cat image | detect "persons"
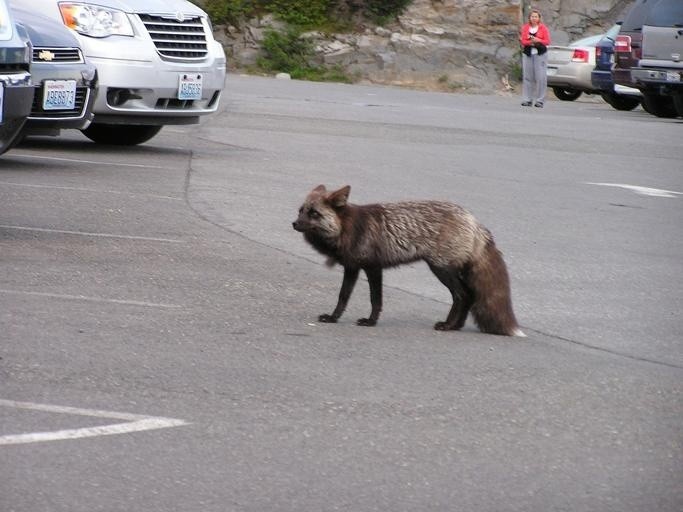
[519,10,551,108]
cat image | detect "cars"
[546,0,682,118]
[0,0,226,157]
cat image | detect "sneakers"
[521,101,544,108]
